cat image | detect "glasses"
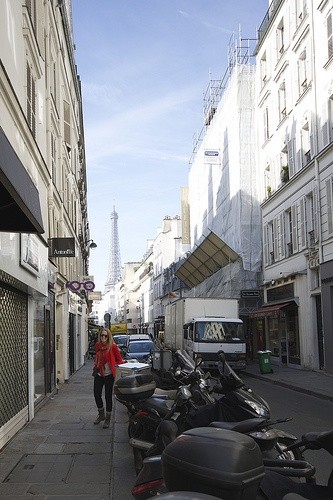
[101,335,108,337]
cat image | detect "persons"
[93,328,124,428]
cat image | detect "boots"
[102,413,111,429]
[93,406,105,425]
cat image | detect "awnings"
[254,301,295,317]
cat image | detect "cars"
[113,334,157,360]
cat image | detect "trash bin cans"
[257,350,273,374]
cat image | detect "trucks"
[164,296,247,375]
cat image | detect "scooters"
[114,348,333,500]
